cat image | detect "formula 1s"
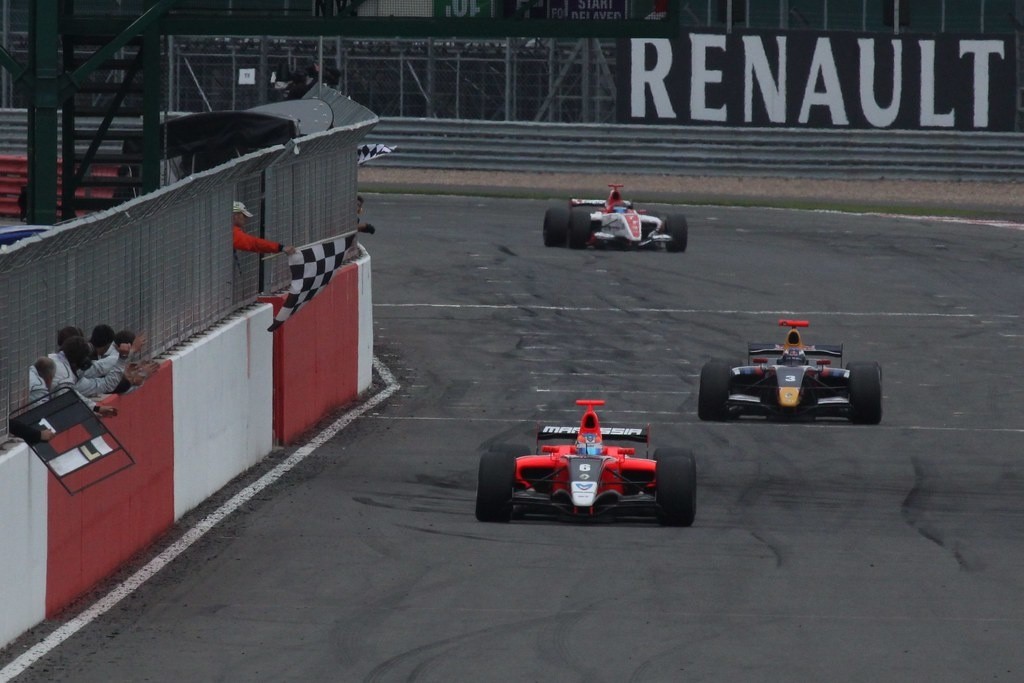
[542,181,690,255]
[690,318,886,425]
[473,399,702,529]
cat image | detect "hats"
[232,201,253,217]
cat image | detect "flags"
[266,230,360,332]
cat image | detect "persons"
[114,330,161,377]
[56,326,94,372]
[47,335,119,418]
[358,194,376,234]
[86,324,145,393]
[9,418,56,442]
[28,356,57,410]
[613,200,627,213]
[574,434,602,456]
[233,199,296,256]
[777,347,806,365]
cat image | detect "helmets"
[608,201,628,213]
[576,433,603,456]
[784,347,807,365]
